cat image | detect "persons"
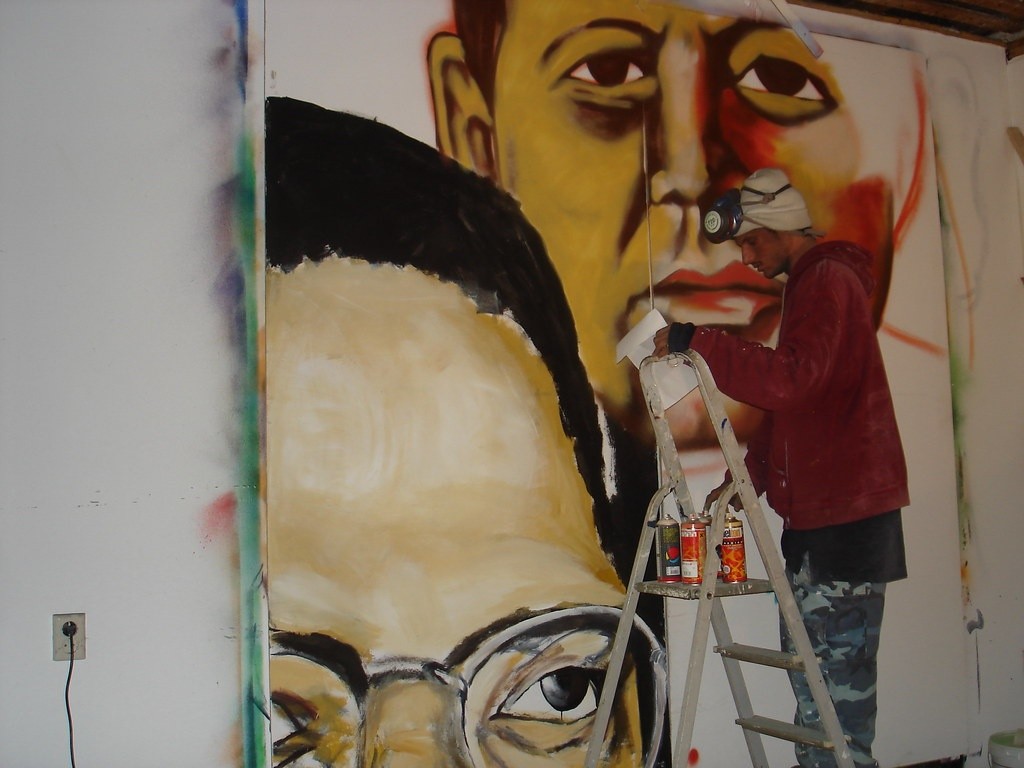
[650,168,911,768]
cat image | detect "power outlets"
[52,613,86,661]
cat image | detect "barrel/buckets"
[987,729,1024,768]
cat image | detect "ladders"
[583,349,856,768]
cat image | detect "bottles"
[721,513,748,584]
[680,510,722,585]
[654,513,682,584]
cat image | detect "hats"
[732,169,812,237]
